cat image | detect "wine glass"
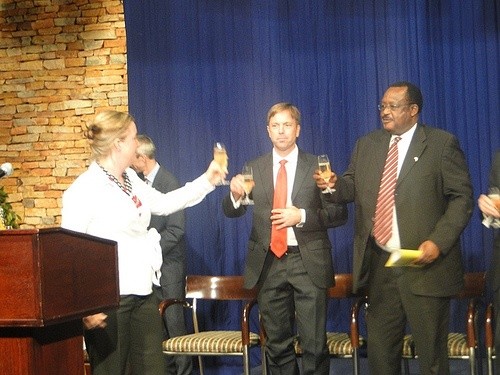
[213,142,229,186]
[317,155,336,194]
[487,187,500,228]
[240,166,254,205]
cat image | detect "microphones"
[0,163,13,178]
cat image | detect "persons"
[477,150,500,375]
[131,135,193,374]
[313,82,474,375]
[222,102,349,375]
[60,111,229,375]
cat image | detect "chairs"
[158,274,500,375]
